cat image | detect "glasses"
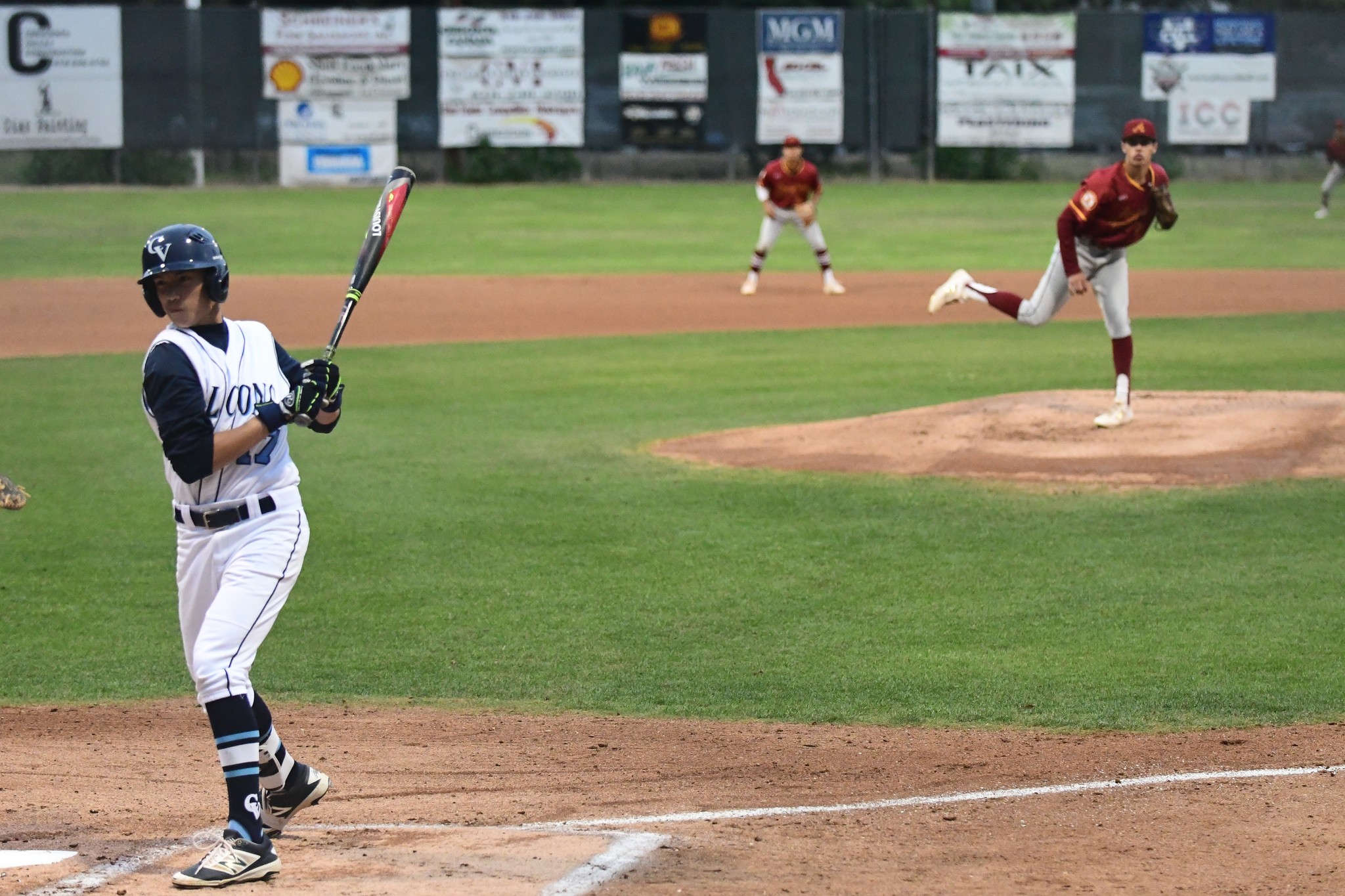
[1127,138,1151,146]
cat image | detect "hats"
[1124,119,1156,142]
[785,136,800,148]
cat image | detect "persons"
[136,223,343,888]
[1313,120,1345,218]
[739,136,847,295]
[0,474,30,510]
[929,119,1177,429]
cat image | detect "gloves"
[254,374,330,433]
[300,360,344,412]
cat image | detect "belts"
[175,496,275,529]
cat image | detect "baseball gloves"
[1150,184,1178,229]
[0,475,27,510]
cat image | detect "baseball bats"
[293,163,416,427]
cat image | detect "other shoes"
[740,273,758,294]
[822,282,844,295]
[1317,207,1329,219]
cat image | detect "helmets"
[137,225,229,317]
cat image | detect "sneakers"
[172,830,282,888]
[928,270,972,315]
[258,763,331,836]
[1094,403,1133,427]
